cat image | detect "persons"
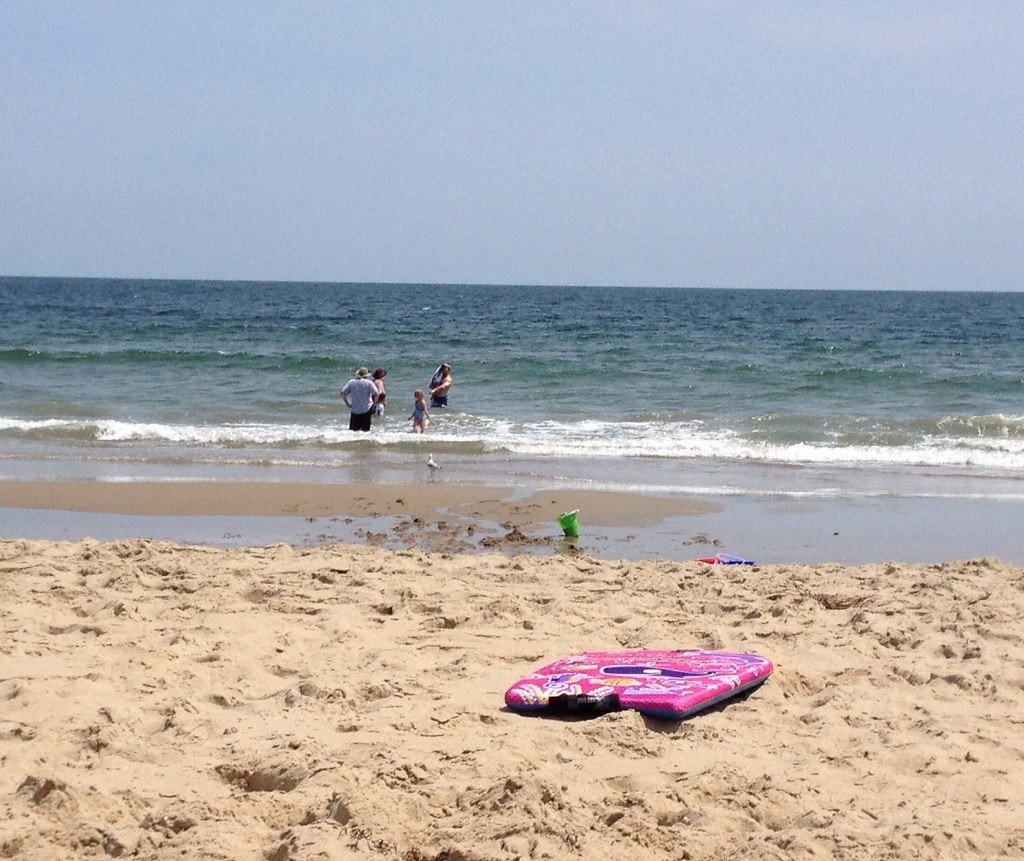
[375,393,386,416]
[341,367,379,431]
[372,368,387,394]
[429,362,453,408]
[408,389,434,434]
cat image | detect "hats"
[373,368,386,379]
[355,366,372,378]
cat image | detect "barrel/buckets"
[555,509,579,538]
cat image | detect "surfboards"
[502,646,775,723]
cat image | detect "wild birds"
[427,453,442,474]
